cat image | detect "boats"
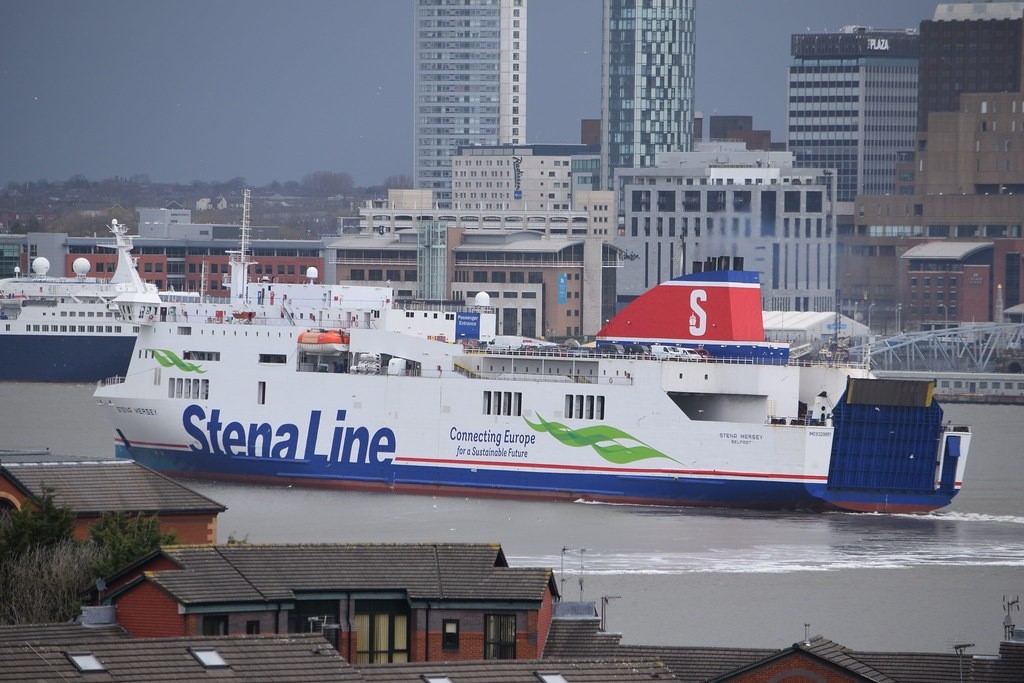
[89,184,973,519]
[0,216,166,380]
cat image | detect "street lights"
[898,303,903,334]
[895,307,901,337]
[868,302,876,330]
[937,304,949,352]
